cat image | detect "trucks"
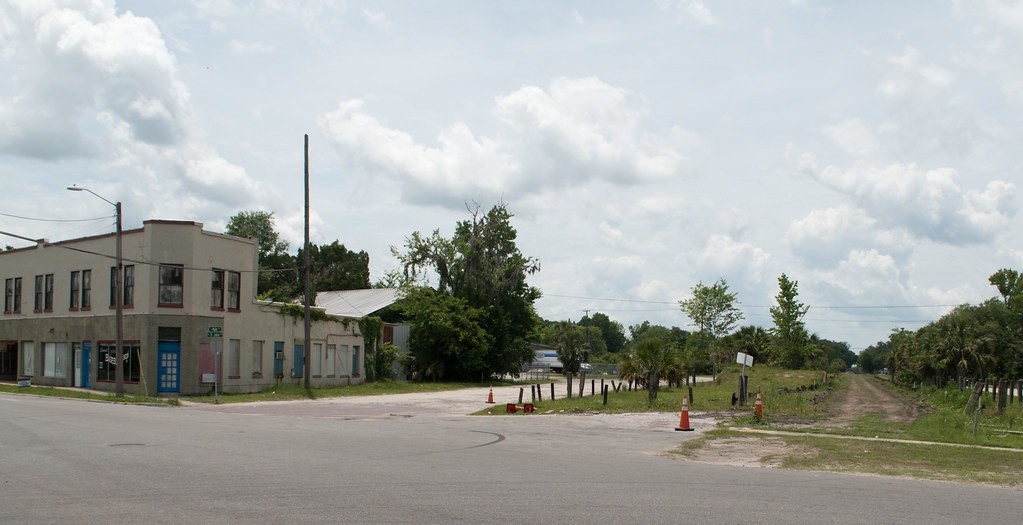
[879,368,890,374]
[527,348,593,373]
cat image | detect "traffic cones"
[505,403,524,412]
[485,384,495,403]
[753,390,764,418]
[522,404,538,414]
[674,393,694,432]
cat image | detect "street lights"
[68,184,125,397]
